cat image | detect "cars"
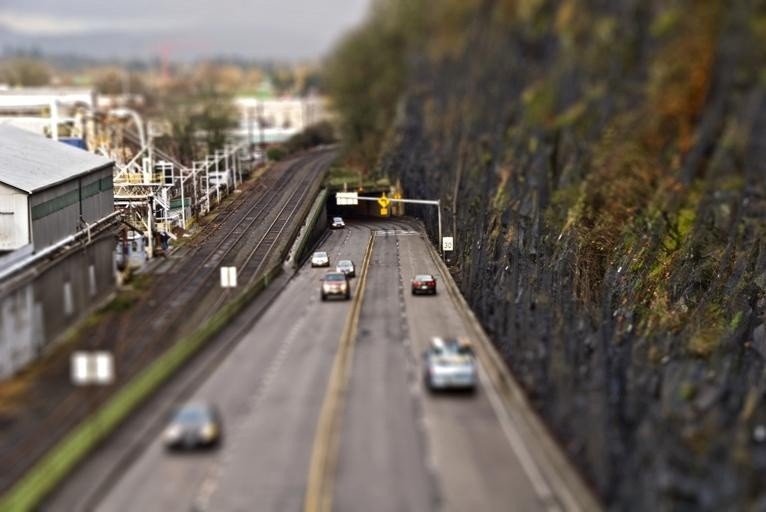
[319,271,350,299]
[164,402,218,453]
[424,339,476,394]
[411,274,436,294]
[331,216,346,229]
[334,259,355,276]
[311,251,329,268]
